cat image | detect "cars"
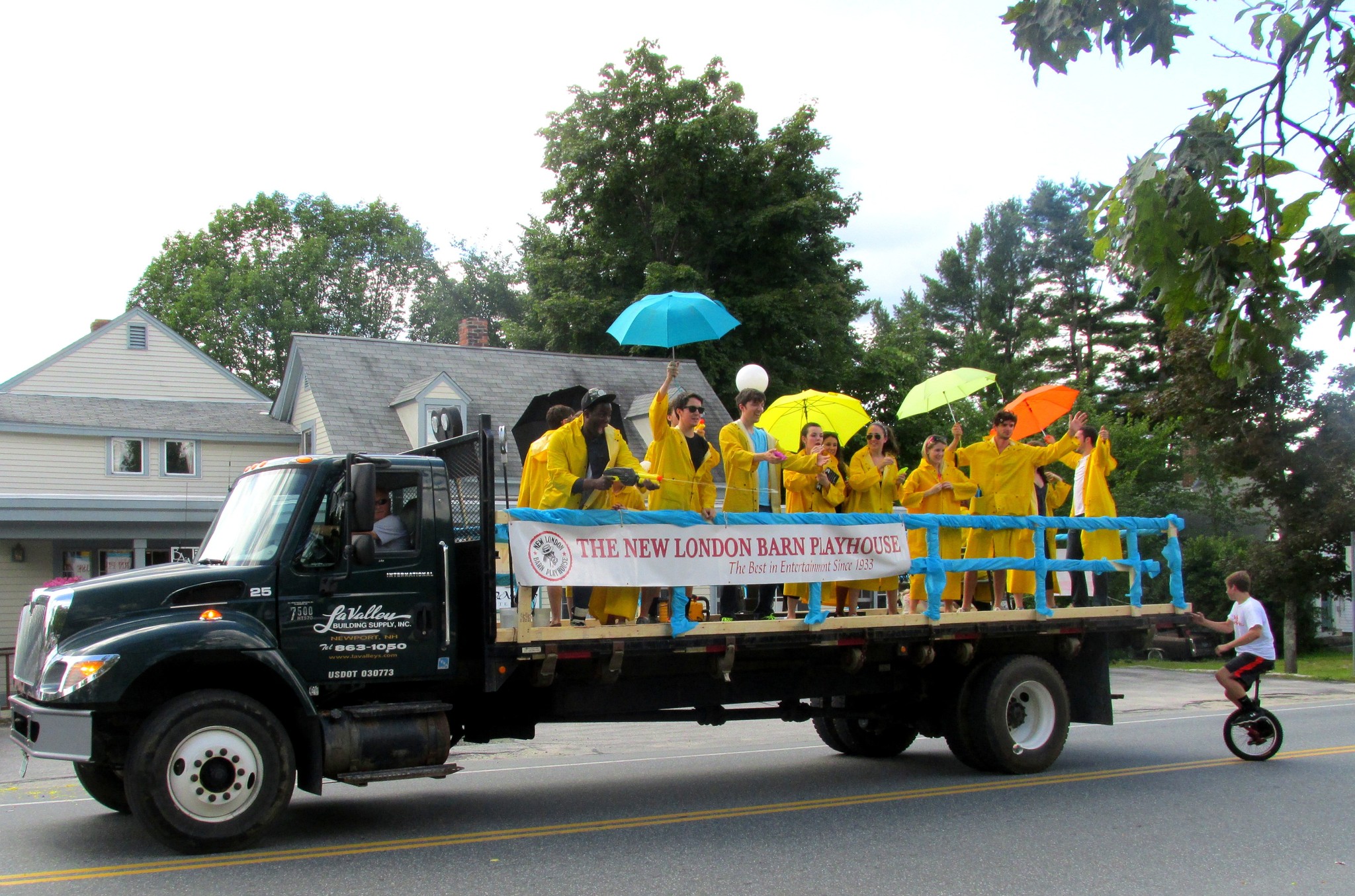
[1106,624,1217,662]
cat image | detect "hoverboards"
[1223,674,1283,762]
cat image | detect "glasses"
[682,406,705,414]
[866,435,885,440]
[926,436,947,445]
[823,431,838,437]
[375,498,390,505]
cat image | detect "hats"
[581,387,616,411]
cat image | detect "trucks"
[7,407,1196,855]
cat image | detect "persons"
[1189,570,1275,743]
[523,386,1125,626]
[309,488,411,554]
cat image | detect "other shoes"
[550,622,562,627]
[993,605,1008,610]
[887,611,900,615]
[849,613,857,616]
[957,608,966,612]
[1051,606,1059,608]
[721,617,733,622]
[635,614,655,624]
[1015,607,1024,610]
[1067,603,1074,608]
[762,613,777,620]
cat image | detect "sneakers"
[1247,725,1274,745]
[1231,709,1265,725]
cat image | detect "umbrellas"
[989,384,1080,442]
[607,291,741,384]
[895,367,1004,448]
[753,389,872,454]
